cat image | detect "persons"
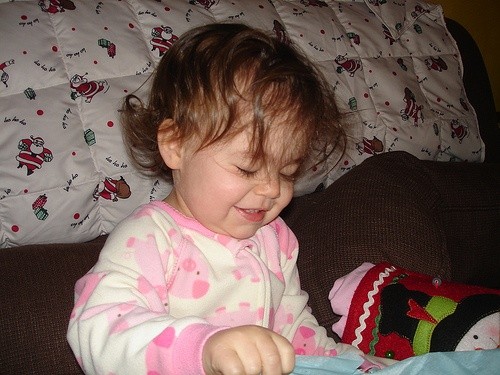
[66,20,383,375]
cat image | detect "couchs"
[1,18,500,375]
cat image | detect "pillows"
[285,148,451,345]
